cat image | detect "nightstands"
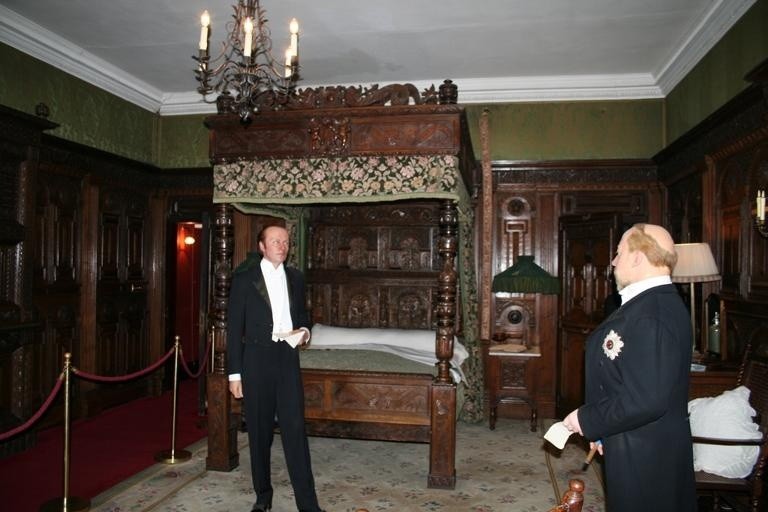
[485,347,544,431]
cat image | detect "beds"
[202,78,484,494]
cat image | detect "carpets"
[86,415,604,512]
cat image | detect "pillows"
[308,319,460,354]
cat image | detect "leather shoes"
[250,491,272,511]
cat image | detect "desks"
[675,363,739,400]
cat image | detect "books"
[488,344,527,352]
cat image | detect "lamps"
[490,252,559,351]
[664,241,722,352]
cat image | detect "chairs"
[675,319,767,510]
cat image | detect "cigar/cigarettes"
[585,445,597,465]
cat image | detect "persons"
[563,222,698,510]
[225,225,326,511]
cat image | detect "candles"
[284,47,294,77]
[753,186,767,224]
[289,16,300,56]
[199,7,209,52]
[243,14,254,56]
